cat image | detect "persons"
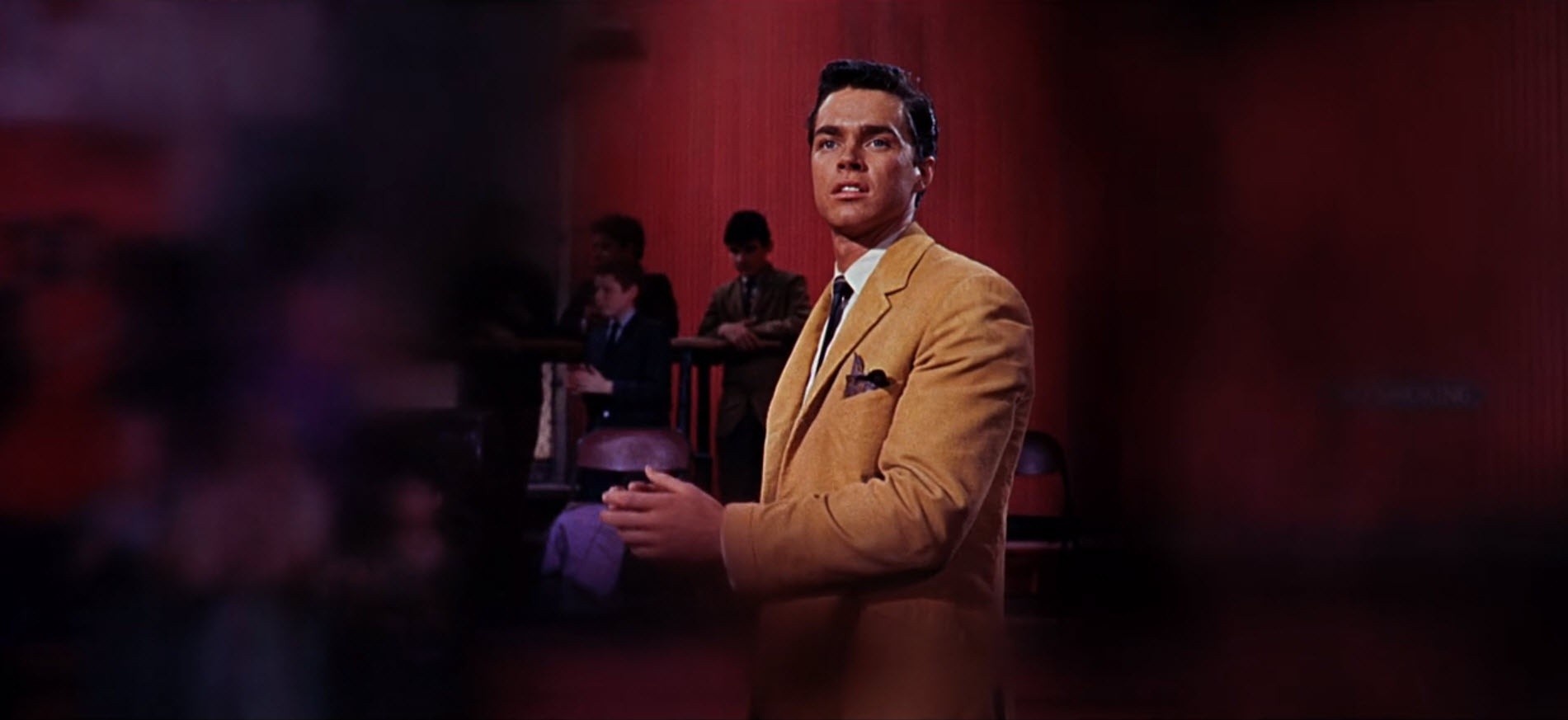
[598,60,1035,720]
[435,206,556,496]
[699,210,809,501]
[552,212,678,431]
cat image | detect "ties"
[608,318,622,347]
[817,275,852,375]
[744,281,755,315]
